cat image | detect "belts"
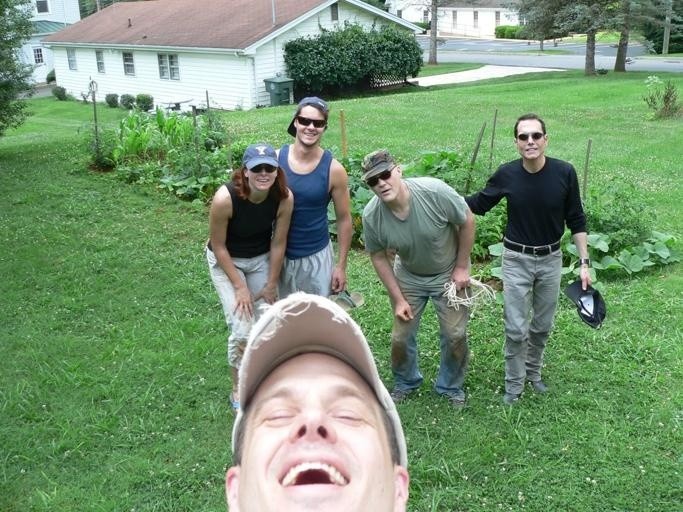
[502,239,561,257]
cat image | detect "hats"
[360,149,396,181]
[229,290,409,471]
[287,96,329,138]
[242,143,279,170]
[563,279,607,331]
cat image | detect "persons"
[273,95,353,310]
[361,147,475,403]
[205,143,294,411]
[225,291,411,511]
[460,113,593,405]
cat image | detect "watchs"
[579,258,590,264]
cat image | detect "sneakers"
[389,385,412,405]
[527,378,546,392]
[503,392,520,405]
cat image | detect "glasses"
[251,165,276,173]
[296,114,326,128]
[518,132,543,140]
[366,164,397,187]
